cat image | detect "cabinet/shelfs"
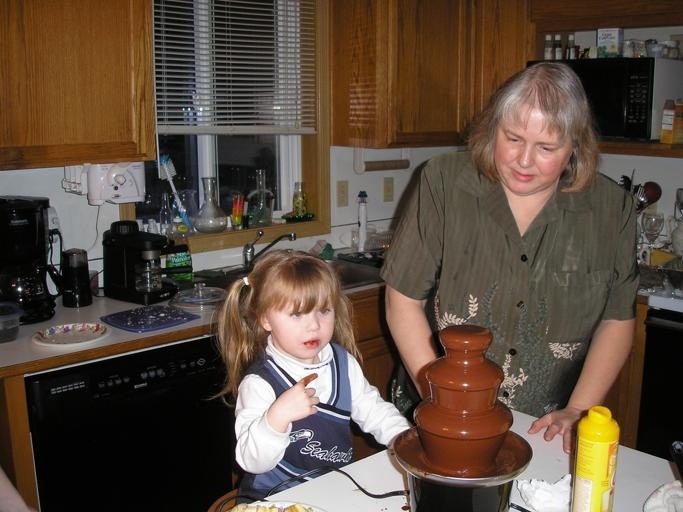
[602,294,650,448]
[330,0,532,149]
[0,0,155,171]
[533,1,683,158]
[331,283,401,459]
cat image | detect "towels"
[642,480,683,512]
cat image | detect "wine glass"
[640,210,665,251]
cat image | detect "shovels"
[636,180,661,212]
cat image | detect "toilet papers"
[358,202,367,252]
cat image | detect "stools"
[207,489,237,512]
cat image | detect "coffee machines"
[100,220,179,305]
[0,194,56,328]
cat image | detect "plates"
[31,322,110,348]
[283,213,315,223]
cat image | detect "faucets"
[243,230,296,268]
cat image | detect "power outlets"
[48,206,59,240]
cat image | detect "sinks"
[321,260,381,295]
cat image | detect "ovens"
[633,315,683,471]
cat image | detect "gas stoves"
[649,285,683,315]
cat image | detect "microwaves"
[526,58,683,144]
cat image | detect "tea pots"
[45,247,92,310]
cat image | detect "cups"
[352,230,377,251]
[230,194,244,229]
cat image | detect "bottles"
[291,181,308,218]
[623,37,683,58]
[147,218,159,235]
[196,174,227,233]
[159,193,173,236]
[251,170,273,225]
[544,33,577,60]
[173,189,198,235]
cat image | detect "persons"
[378,62,640,455]
[205,250,414,507]
